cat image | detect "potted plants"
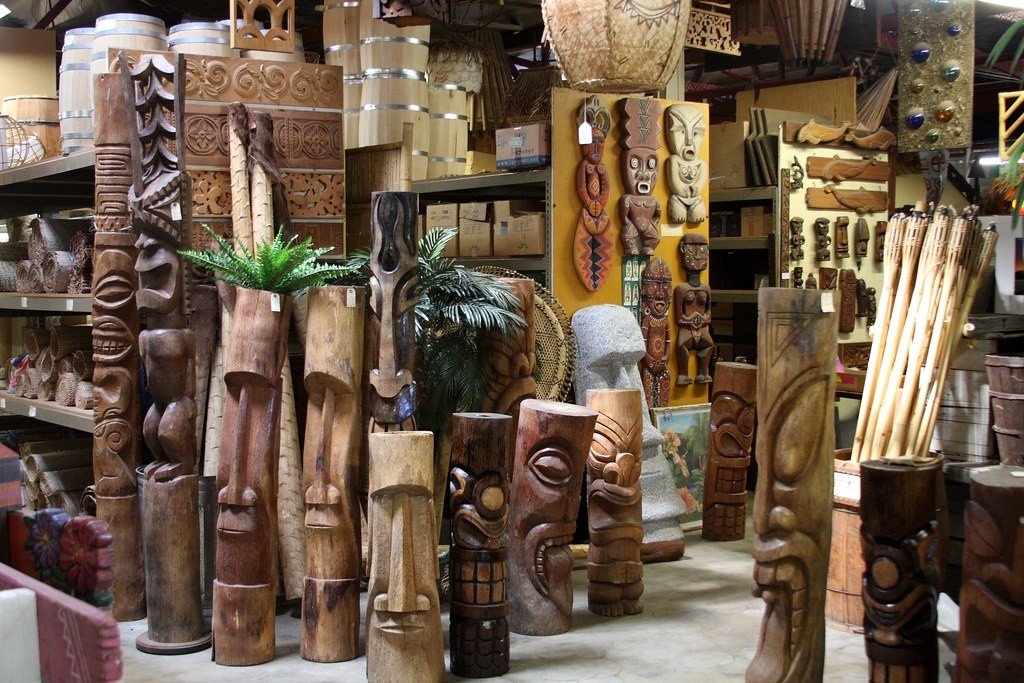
[176,218,353,666]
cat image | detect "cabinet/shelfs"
[0,151,96,435]
[710,185,780,302]
[411,168,551,292]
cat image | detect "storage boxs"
[495,123,551,170]
[709,206,773,361]
[493,200,547,257]
[457,202,495,257]
[426,203,458,258]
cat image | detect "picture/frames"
[649,402,712,532]
